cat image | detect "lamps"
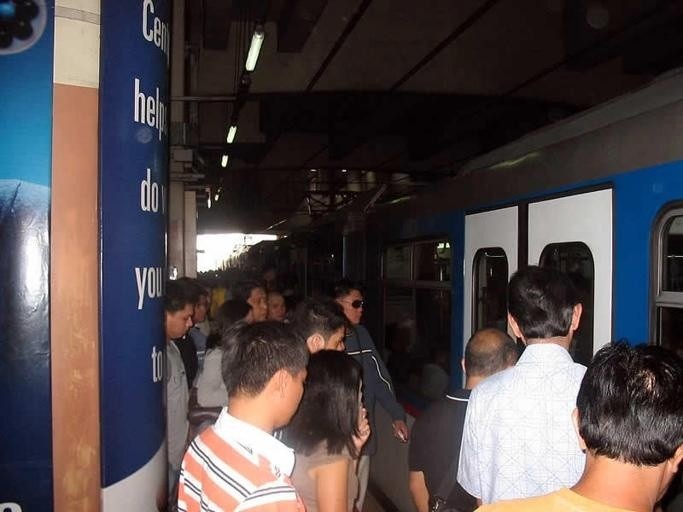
[221,27,265,170]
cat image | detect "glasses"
[340,299,365,307]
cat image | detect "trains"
[218,67,683,393]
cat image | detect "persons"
[410,266,683,512]
[164,262,409,511]
